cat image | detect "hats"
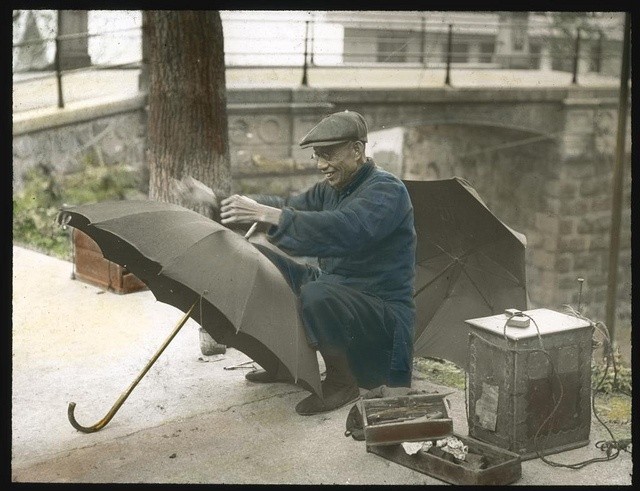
[299,111,368,149]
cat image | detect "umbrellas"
[57,199,325,434]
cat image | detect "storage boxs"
[72,228,151,295]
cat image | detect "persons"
[172,110,415,417]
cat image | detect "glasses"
[310,152,332,161]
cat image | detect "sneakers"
[295,376,361,416]
[245,367,288,382]
